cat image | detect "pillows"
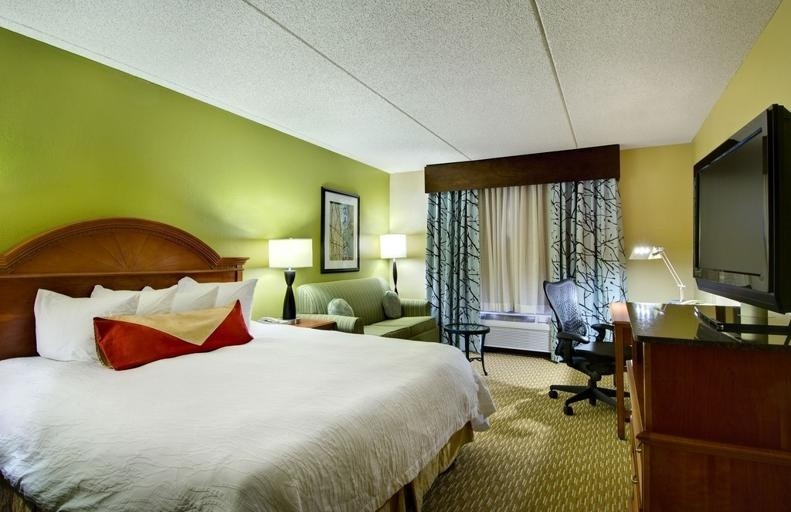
[327,298,354,316]
[382,290,402,319]
[32,277,257,363]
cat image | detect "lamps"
[629,244,697,306]
[268,238,313,320]
[380,235,407,298]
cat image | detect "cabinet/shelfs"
[629,304,791,512]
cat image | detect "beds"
[0,217,496,512]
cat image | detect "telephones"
[258,317,288,325]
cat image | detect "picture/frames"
[320,186,360,275]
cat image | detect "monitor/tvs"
[693,103,791,331]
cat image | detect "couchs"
[298,277,440,343]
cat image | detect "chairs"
[542,278,631,415]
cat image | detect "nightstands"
[293,319,337,331]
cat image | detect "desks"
[610,303,629,439]
